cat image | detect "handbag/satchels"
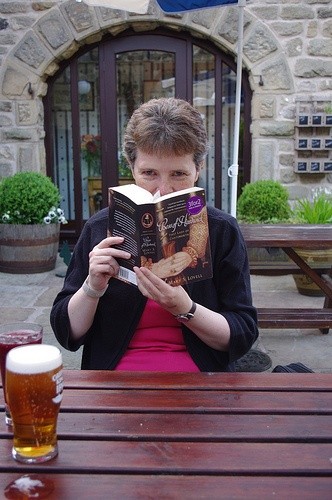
[272,362,316,374]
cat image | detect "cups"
[5,344,64,464]
[0,322,43,427]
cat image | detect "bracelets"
[81,275,109,298]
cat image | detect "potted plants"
[276,185,332,298]
[236,179,297,277]
[0,171,69,275]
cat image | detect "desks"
[0,368,332,500]
[238,221,332,336]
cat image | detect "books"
[106,183,213,292]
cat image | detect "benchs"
[256,307,332,329]
[248,260,332,277]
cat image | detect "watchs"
[172,301,197,325]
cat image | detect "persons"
[50,98,259,372]
